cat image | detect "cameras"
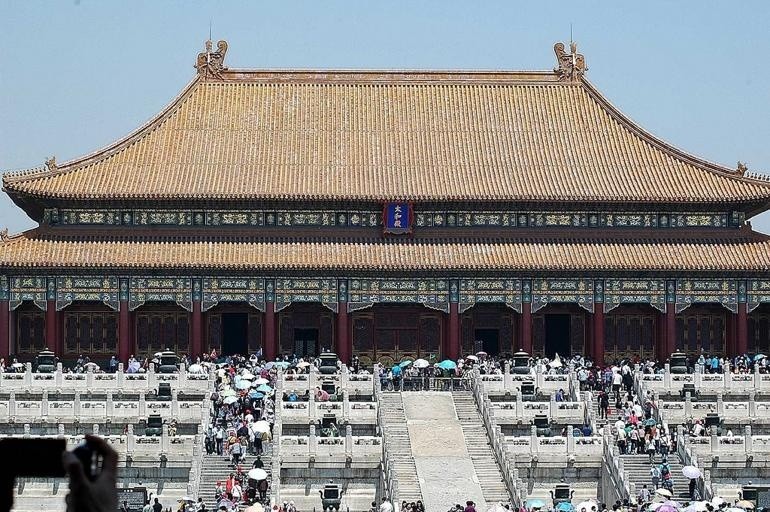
[0,437,100,477]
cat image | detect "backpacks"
[662,463,669,476]
[653,468,659,476]
